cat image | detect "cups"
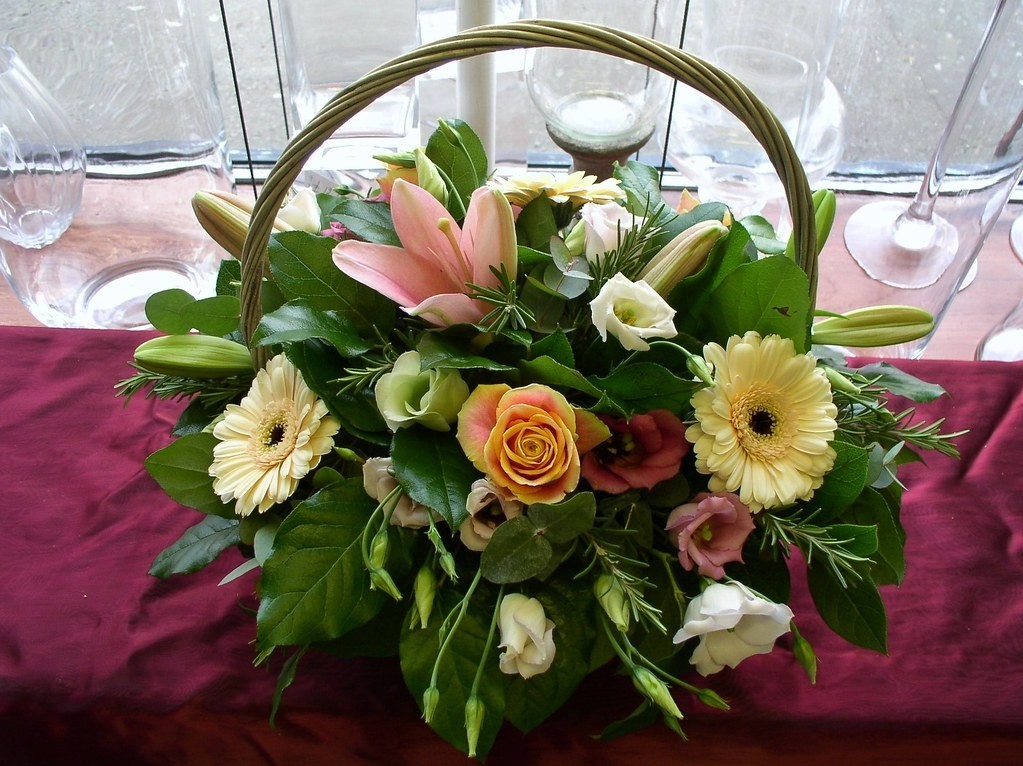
[0,44,85,250]
[0,0,238,332]
[278,0,417,171]
[519,0,1023,360]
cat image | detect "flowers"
[103,108,963,762]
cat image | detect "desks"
[0,324,1023,765]
